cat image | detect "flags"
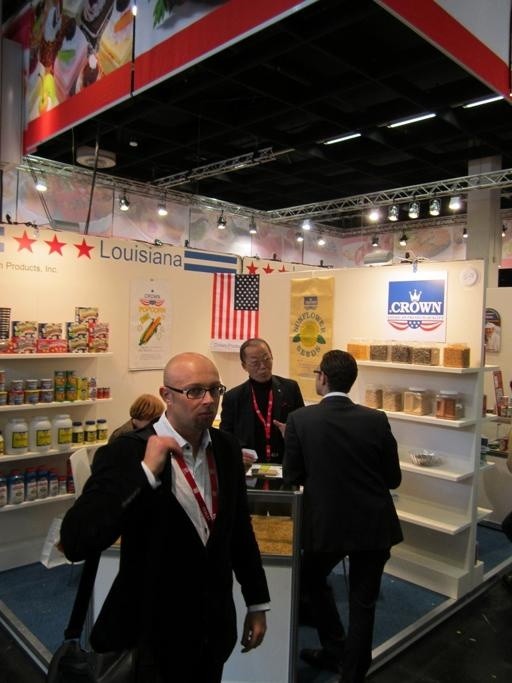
[210,271,259,354]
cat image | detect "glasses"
[166,385,228,399]
[313,368,322,374]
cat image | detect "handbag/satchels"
[47,640,136,683]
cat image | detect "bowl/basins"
[409,448,438,466]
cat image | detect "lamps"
[463,228,469,239]
[249,218,256,233]
[448,195,461,210]
[302,219,310,231]
[325,131,361,145]
[463,97,503,108]
[399,234,407,247]
[409,203,420,219]
[372,236,379,246]
[318,235,325,245]
[502,226,507,237]
[430,198,441,215]
[119,192,128,211]
[36,165,47,192]
[296,232,304,241]
[387,112,436,128]
[218,209,226,229]
[388,206,398,222]
[158,198,167,216]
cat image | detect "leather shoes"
[299,647,346,671]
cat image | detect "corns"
[139,318,160,345]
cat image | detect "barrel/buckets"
[29,416,52,453]
[3,417,28,455]
[51,414,75,451]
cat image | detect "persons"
[60,353,271,683]
[219,337,305,515]
[108,394,166,445]
[282,350,403,681]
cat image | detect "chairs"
[69,448,93,501]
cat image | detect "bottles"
[0,414,108,506]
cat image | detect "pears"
[37,72,56,102]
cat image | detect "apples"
[38,97,48,115]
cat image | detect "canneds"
[0,369,110,406]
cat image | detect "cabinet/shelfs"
[484,411,512,459]
[0,352,113,572]
[350,360,478,601]
[472,365,500,591]
